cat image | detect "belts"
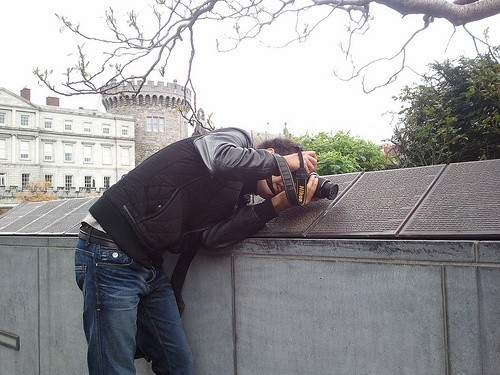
[78,230,118,249]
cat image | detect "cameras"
[291,170,338,200]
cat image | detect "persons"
[74,127,318,375]
